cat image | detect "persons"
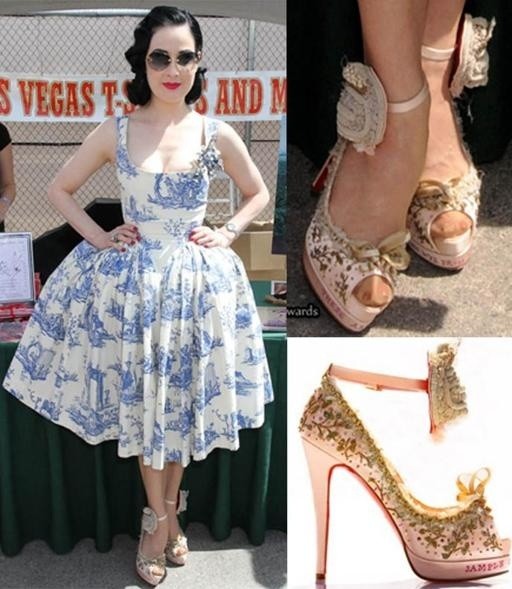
[299,0,502,336]
[1,6,271,587]
[0,119,18,232]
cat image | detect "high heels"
[406,13,497,268]
[301,60,431,335]
[298,343,512,584]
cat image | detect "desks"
[1,280,286,558]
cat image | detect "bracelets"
[0,195,12,207]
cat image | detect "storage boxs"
[219,222,287,281]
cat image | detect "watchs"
[224,222,240,238]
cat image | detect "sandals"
[136,489,190,587]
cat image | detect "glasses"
[144,49,198,72]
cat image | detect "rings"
[110,232,119,242]
